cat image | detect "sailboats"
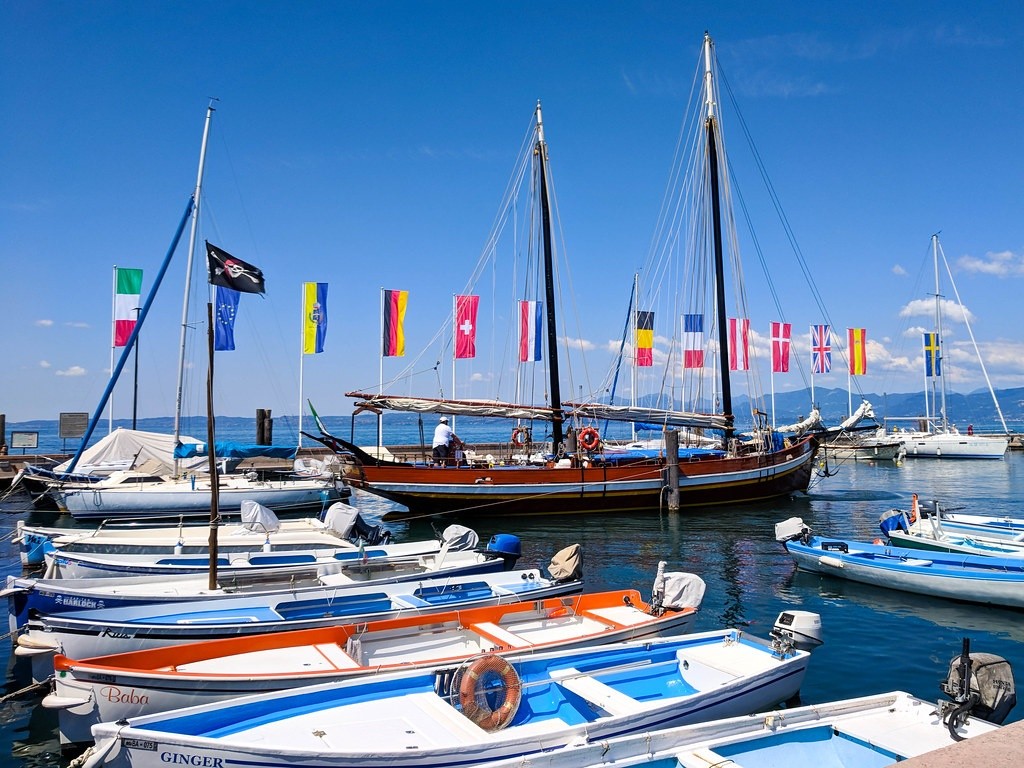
[862,232,1012,458]
[8,86,355,515]
[341,28,874,517]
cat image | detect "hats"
[440,417,449,422]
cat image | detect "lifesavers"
[460,655,520,730]
[512,430,529,446]
[580,428,599,450]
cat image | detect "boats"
[816,435,905,463]
[470,637,1018,768]
[0,502,823,767]
[21,424,245,478]
[770,500,1023,609]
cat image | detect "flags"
[772,322,791,372]
[304,282,328,354]
[519,301,543,362]
[729,319,750,371]
[848,329,866,376]
[384,289,408,357]
[205,239,265,300]
[633,311,655,367]
[684,314,703,368]
[215,286,241,351]
[114,268,143,346]
[812,324,831,373]
[456,295,479,359]
[925,333,941,377]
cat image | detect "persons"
[433,417,463,466]
[968,424,973,436]
